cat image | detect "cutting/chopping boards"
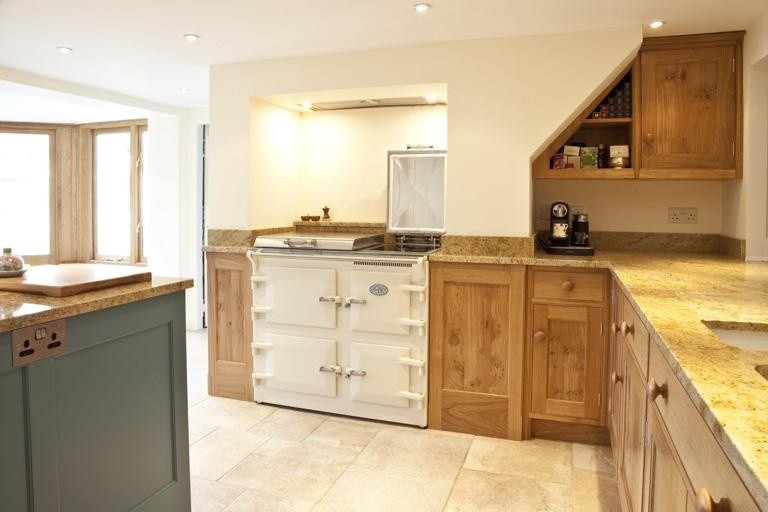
[0,263,153,299]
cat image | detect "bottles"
[597,143,606,168]
[0,248,24,278]
[571,210,589,246]
[322,205,330,221]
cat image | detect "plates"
[0,265,34,278]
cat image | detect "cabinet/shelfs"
[429,261,762,512]
[532,31,745,180]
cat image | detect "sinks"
[755,365,768,381]
[701,320,768,351]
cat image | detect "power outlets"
[668,207,698,224]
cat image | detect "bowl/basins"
[310,216,320,221]
[607,157,630,168]
[300,215,309,221]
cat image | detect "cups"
[552,221,567,236]
